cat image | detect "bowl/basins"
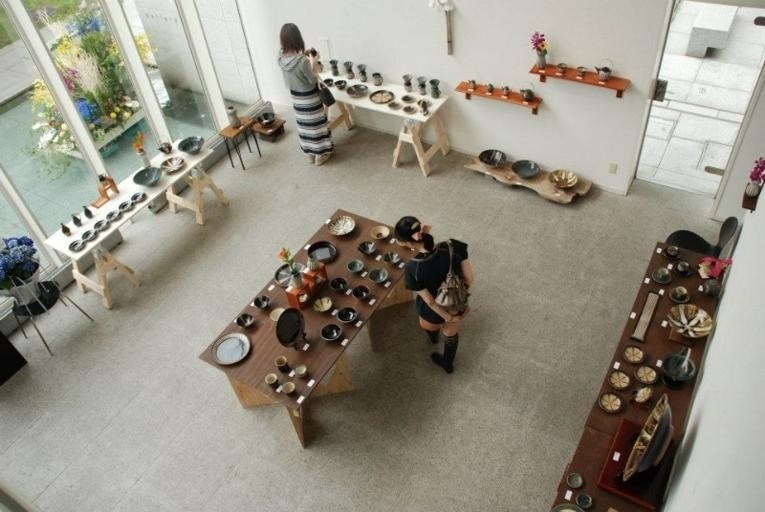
[548,169,579,189]
[478,149,507,169]
[132,133,205,186]
[370,225,390,240]
[256,112,277,128]
[512,160,541,180]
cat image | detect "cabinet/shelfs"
[452,57,630,117]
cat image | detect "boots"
[425,328,458,374]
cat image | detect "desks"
[550,425,660,512]
[251,118,287,143]
[41,137,232,312]
[314,71,453,178]
[195,209,428,451]
[219,114,263,170]
[11,280,94,357]
[583,240,728,449]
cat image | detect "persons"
[277,23,335,166]
[395,216,475,374]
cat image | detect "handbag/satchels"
[314,71,336,108]
[433,239,470,315]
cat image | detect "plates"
[314,59,441,117]
[600,246,719,482]
[211,333,252,366]
[566,473,584,489]
[56,191,147,254]
[576,494,594,509]
[550,503,586,512]
[326,216,356,237]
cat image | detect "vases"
[745,179,761,199]
[306,255,318,272]
[137,151,152,169]
[58,203,95,236]
[536,50,547,69]
[6,261,43,307]
[289,270,303,290]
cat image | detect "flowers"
[0,234,68,320]
[750,156,765,187]
[277,245,299,274]
[25,0,160,163]
[530,30,549,56]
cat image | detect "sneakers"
[305,152,331,167]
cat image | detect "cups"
[576,66,587,76]
[294,364,308,378]
[274,355,288,369]
[282,381,296,394]
[265,373,278,386]
[557,63,567,73]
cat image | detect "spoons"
[235,238,402,347]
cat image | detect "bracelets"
[448,316,453,323]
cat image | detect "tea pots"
[467,77,536,102]
[593,58,614,82]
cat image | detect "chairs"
[665,214,740,260]
